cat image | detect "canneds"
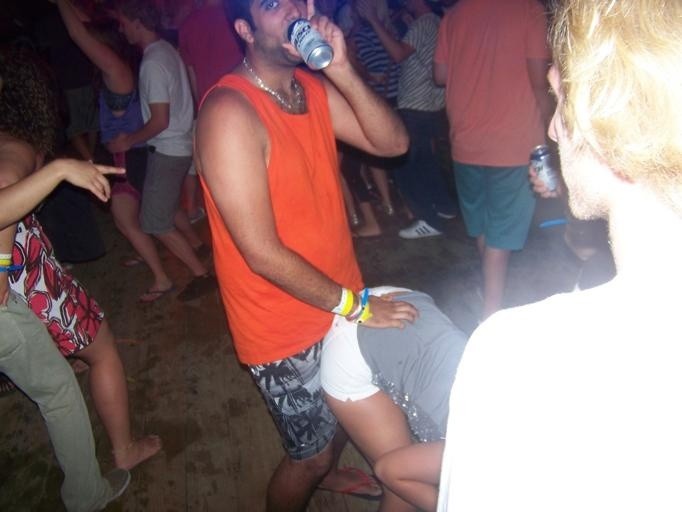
[287,19,333,71]
[530,146,559,193]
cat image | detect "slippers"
[123,258,144,267]
[316,468,383,500]
[139,282,175,302]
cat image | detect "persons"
[314,1,607,324]
[192,1,411,512]
[435,0,682,511]
[2,1,211,512]
[319,285,463,511]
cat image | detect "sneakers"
[104,466,131,502]
[349,204,457,239]
[178,273,217,302]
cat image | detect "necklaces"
[243,56,302,110]
